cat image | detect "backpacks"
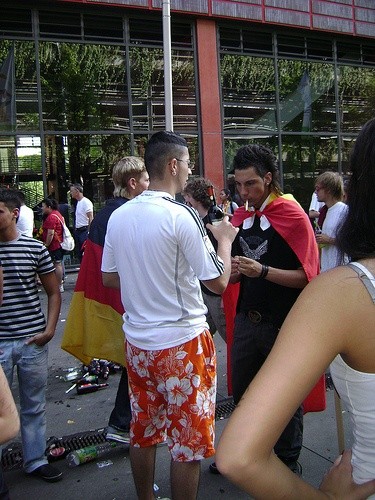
[52,214,75,251]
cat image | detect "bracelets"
[259,265,269,279]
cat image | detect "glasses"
[315,186,324,191]
[168,158,195,168]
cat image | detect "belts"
[236,309,272,321]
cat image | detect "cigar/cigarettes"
[245,198,249,212]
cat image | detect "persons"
[0,156,152,482]
[102,129,238,500]
[180,173,239,344]
[308,170,353,273]
[209,145,325,478]
[216,117,375,500]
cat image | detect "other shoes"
[291,460,302,476]
[209,463,218,474]
[32,464,64,482]
[59,284,64,293]
[105,425,130,443]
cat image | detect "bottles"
[63,358,122,380]
[72,375,98,385]
[45,446,71,462]
[207,185,224,226]
[76,383,109,395]
[313,220,328,248]
[65,441,117,467]
